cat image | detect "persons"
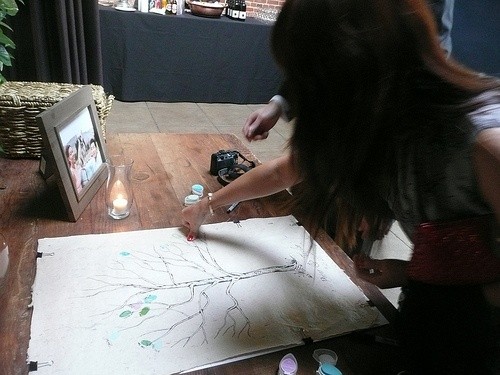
[243,0,455,259]
[181,0,500,375]
[66,139,102,194]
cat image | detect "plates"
[115,7,136,11]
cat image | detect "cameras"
[210,150,238,175]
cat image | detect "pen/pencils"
[226,201,240,214]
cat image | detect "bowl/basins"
[190,3,224,19]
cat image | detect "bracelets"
[208,193,215,215]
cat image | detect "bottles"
[225,0,246,22]
[106,155,134,220]
[149,0,178,15]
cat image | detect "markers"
[350,332,399,347]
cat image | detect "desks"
[99,4,283,104]
[0,132,397,375]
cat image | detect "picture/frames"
[36,86,113,222]
